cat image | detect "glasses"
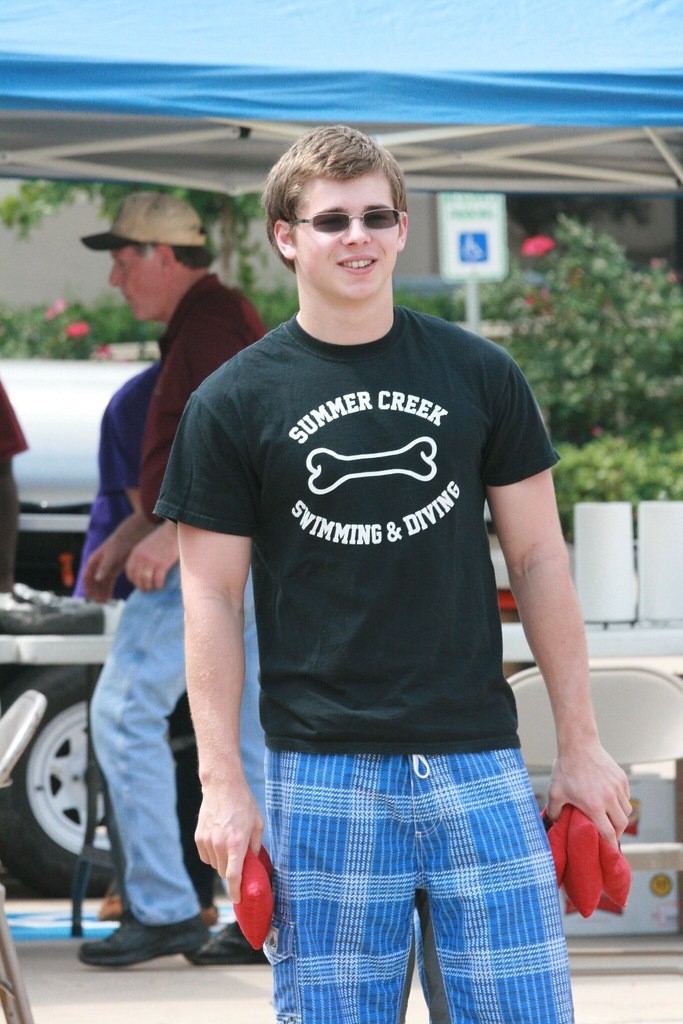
[290,208,400,233]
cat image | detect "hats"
[81,192,209,249]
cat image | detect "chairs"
[495,662,683,938]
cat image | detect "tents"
[0,0,683,195]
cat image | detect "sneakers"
[80,914,209,966]
[184,920,266,966]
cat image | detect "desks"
[0,623,533,937]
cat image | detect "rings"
[145,571,152,577]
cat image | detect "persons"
[152,124,631,1023]
[0,378,28,594]
[84,191,274,968]
[73,364,219,931]
